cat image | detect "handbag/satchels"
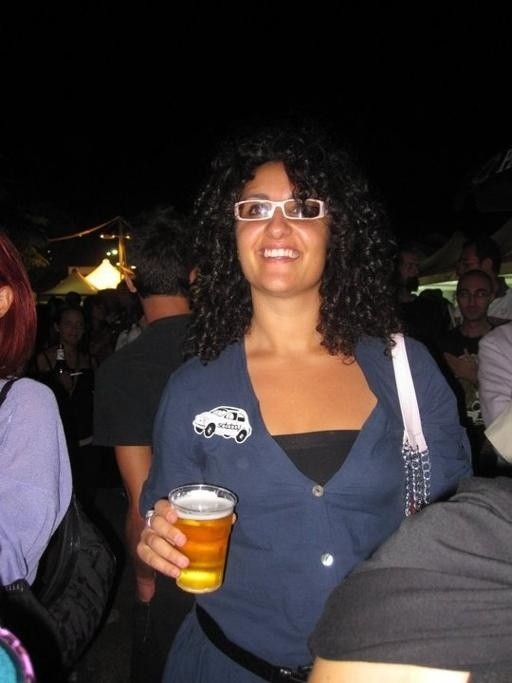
[387,326,477,519]
[1,372,120,683]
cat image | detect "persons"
[0,209,511,683]
[133,125,479,681]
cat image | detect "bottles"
[54,343,68,376]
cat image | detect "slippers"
[232,198,329,221]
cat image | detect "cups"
[167,483,240,594]
